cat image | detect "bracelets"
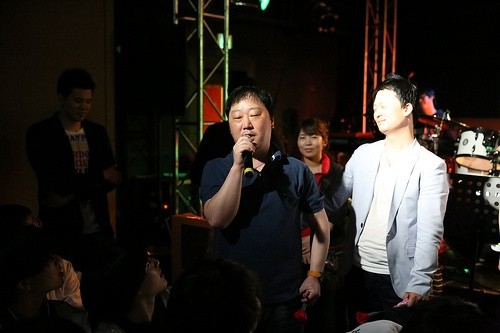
[307,271,322,278]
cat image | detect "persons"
[200,85,331,333]
[0,68,444,333]
[324,76,451,333]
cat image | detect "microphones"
[242,150,254,179]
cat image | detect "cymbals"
[430,114,471,128]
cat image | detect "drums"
[455,127,500,172]
